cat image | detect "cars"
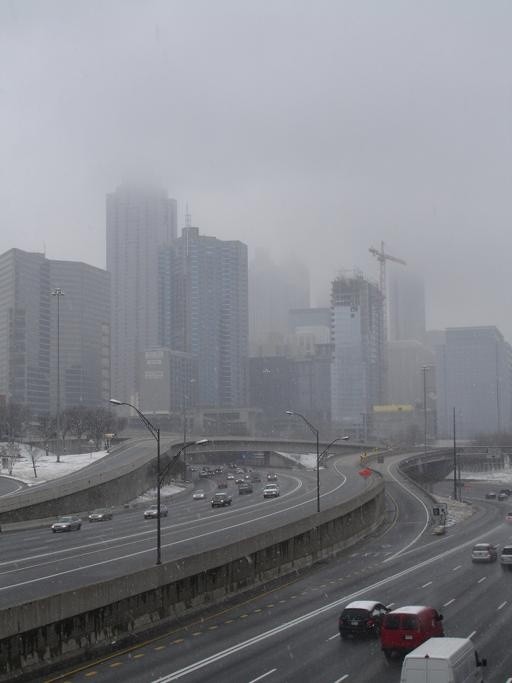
[88,508,114,522]
[336,598,392,639]
[51,515,83,533]
[142,504,170,518]
[484,486,512,503]
[183,460,283,508]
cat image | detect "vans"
[376,604,445,665]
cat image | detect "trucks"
[398,635,488,683]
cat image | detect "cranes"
[369,237,410,403]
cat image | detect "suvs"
[471,542,498,562]
[499,545,512,567]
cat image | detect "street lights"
[420,363,431,453]
[285,410,350,512]
[48,286,65,462]
[179,379,200,483]
[108,397,208,566]
